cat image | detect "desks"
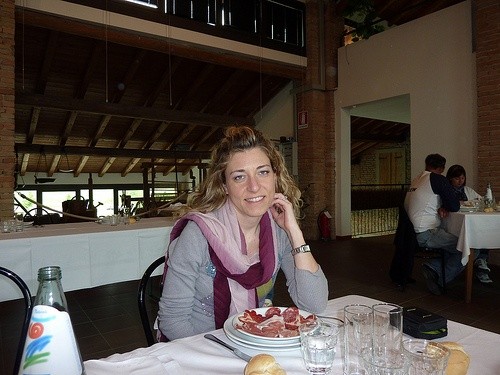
[0,216,177,303]
[442,212,500,305]
[83,294,500,375]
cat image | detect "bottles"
[486,183,493,204]
[17,265,86,375]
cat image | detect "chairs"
[389,200,458,296]
[138,257,164,345]
[0,266,32,375]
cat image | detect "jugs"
[63,195,103,223]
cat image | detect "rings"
[284,196,288,199]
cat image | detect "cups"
[343,304,374,375]
[0,212,24,233]
[297,321,340,375]
[103,214,121,225]
[401,339,451,375]
[371,303,403,375]
[358,345,407,375]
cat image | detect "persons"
[404,153,465,294]
[439,165,491,283]
[154,126,328,342]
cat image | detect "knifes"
[204,333,252,362]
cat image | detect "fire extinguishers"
[318,207,331,240]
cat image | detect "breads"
[244,354,287,375]
[426,341,470,375]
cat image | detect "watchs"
[291,245,310,256]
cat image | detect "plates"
[460,207,476,211]
[223,306,319,352]
[23,222,34,228]
